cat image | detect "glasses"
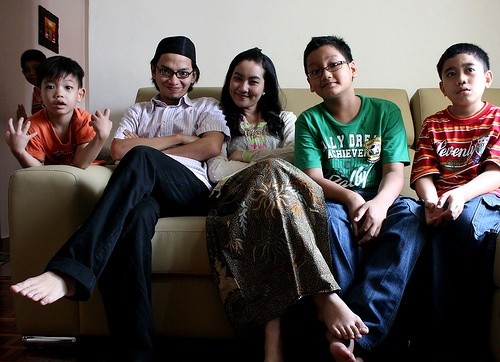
[307,61,349,79]
[154,65,194,79]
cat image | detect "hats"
[154,36,196,62]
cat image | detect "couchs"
[8,87,500,346]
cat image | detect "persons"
[410,43,499,362]
[10,36,232,362]
[17,49,47,122]
[294,36,424,362]
[206,47,372,362]
[5,56,113,171]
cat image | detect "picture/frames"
[38,5,59,53]
